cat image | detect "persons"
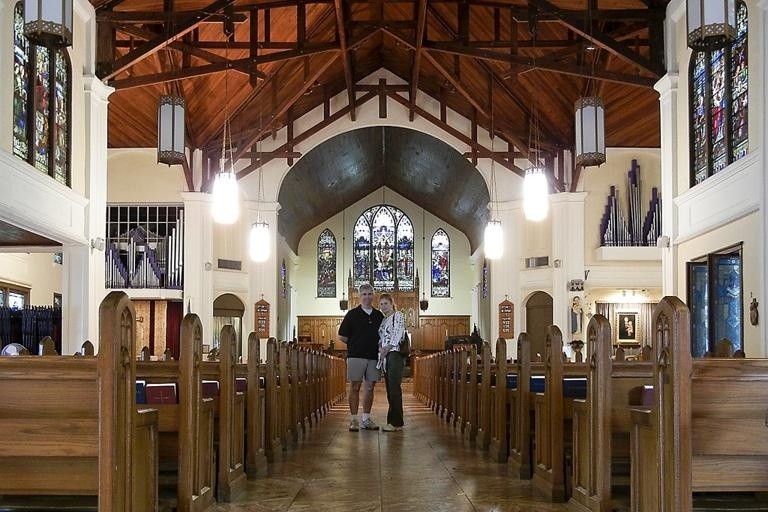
[626,321,633,336]
[620,316,634,340]
[573,297,585,335]
[377,292,406,431]
[337,284,384,431]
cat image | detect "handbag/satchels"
[393,311,411,358]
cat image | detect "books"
[201,379,220,399]
[236,377,247,395]
[564,378,589,399]
[258,374,292,389]
[449,370,545,393]
[147,383,178,405]
[641,384,654,405]
[135,380,148,403]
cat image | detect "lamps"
[684,0,739,51]
[338,193,349,313]
[482,60,506,264]
[156,32,185,170]
[418,205,430,315]
[21,0,73,52]
[573,39,608,171]
[208,27,242,227]
[245,43,276,265]
[89,236,105,253]
[584,263,593,282]
[522,26,549,228]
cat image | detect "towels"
[375,352,387,374]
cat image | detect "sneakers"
[349,419,359,432]
[360,418,380,430]
[382,423,404,432]
